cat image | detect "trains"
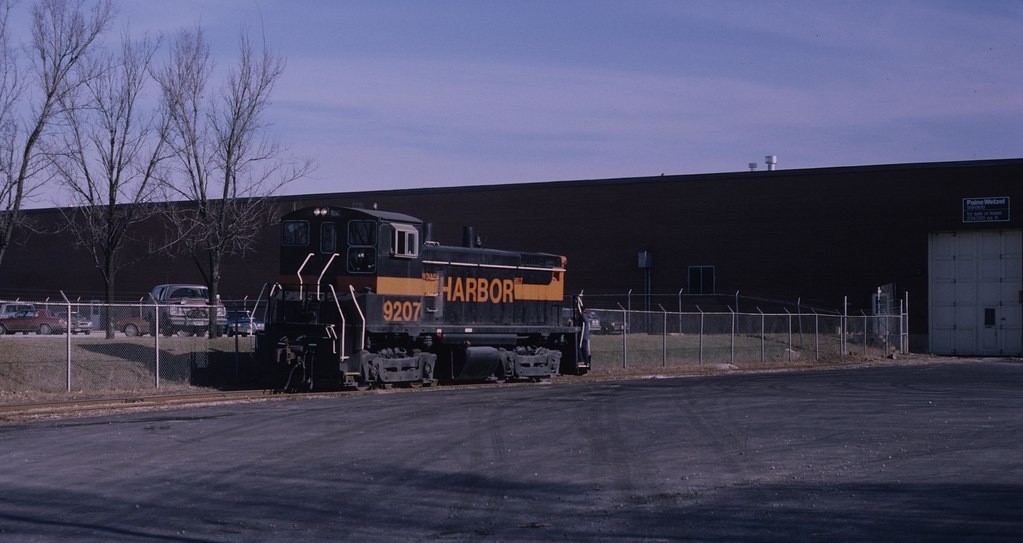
[266,204,593,392]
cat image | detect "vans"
[148,284,229,337]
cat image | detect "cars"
[226,310,266,335]
[0,303,93,335]
[117,318,151,337]
